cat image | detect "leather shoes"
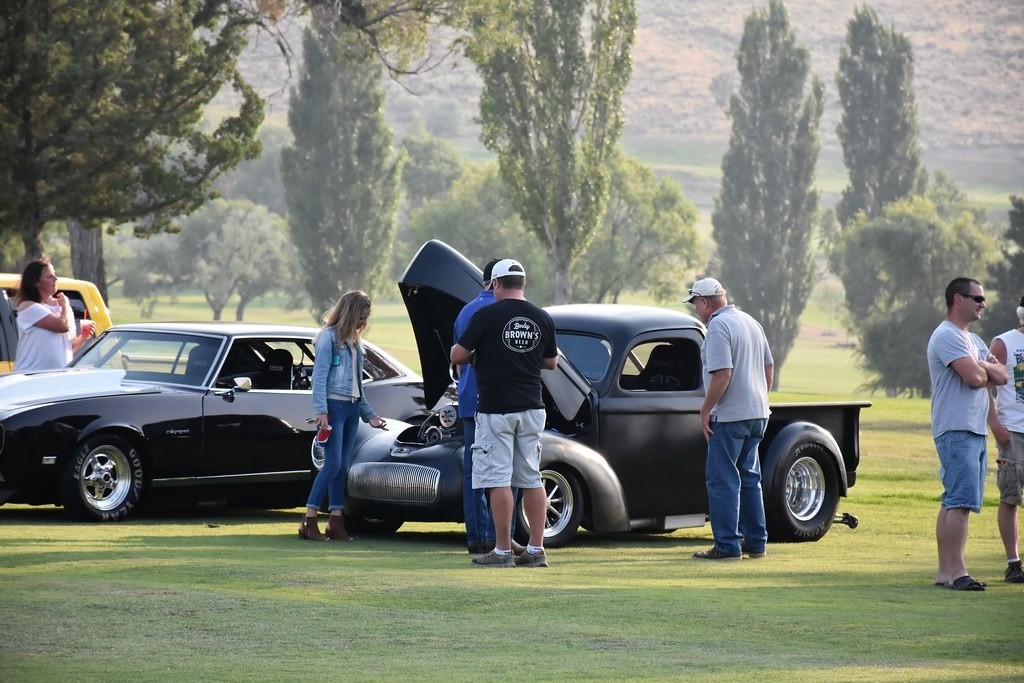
[740,539,766,558]
[693,548,742,560]
[467,537,528,556]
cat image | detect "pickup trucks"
[311,238,873,551]
[0,272,189,375]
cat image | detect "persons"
[682,278,774,560]
[298,289,389,542]
[450,259,558,567]
[985,296,1024,584]
[14,260,94,375]
[927,277,1008,591]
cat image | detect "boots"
[299,514,330,541]
[325,515,354,541]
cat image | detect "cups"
[80,320,93,339]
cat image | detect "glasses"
[689,290,702,298]
[959,294,986,303]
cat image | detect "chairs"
[182,343,218,381]
[253,349,293,389]
[630,345,684,393]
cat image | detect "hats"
[484,259,526,291]
[483,258,501,280]
[682,278,725,304]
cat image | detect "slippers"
[934,582,986,587]
[944,576,984,591]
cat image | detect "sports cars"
[0,320,437,526]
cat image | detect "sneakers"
[1005,560,1024,582]
[514,550,547,566]
[471,551,516,567]
